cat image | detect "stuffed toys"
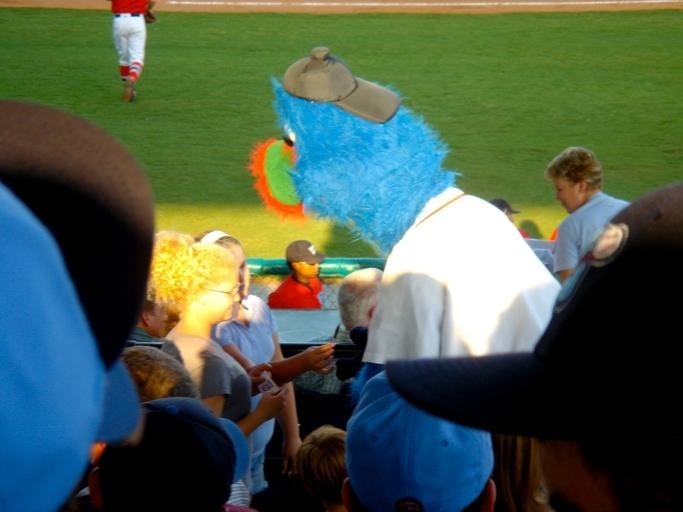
[247,45,562,402]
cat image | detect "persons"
[387,186,682,510]
[109,1,156,103]
[489,198,530,239]
[338,266,384,348]
[88,231,348,512]
[546,147,630,281]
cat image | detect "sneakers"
[123,78,133,103]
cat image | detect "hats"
[97,380,250,509]
[490,200,520,215]
[287,240,325,263]
[385,180,681,446]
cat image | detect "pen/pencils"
[324,324,341,360]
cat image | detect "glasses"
[206,283,241,296]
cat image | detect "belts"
[114,14,142,16]
